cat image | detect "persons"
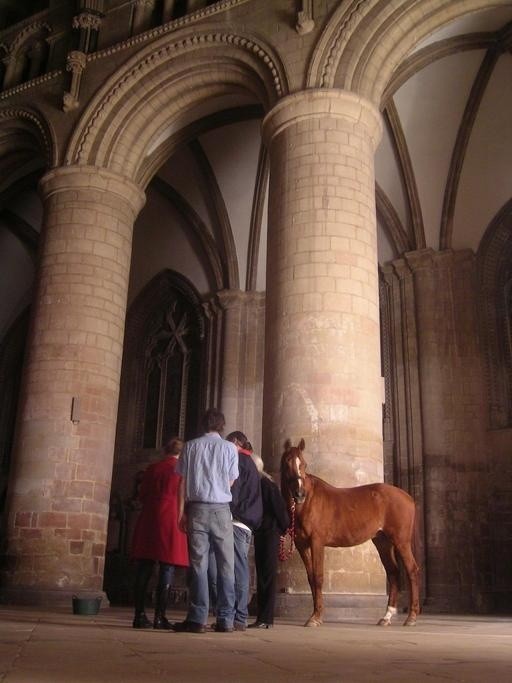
[131,408,292,634]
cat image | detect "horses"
[280,437,422,627]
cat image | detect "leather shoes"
[132,611,273,633]
